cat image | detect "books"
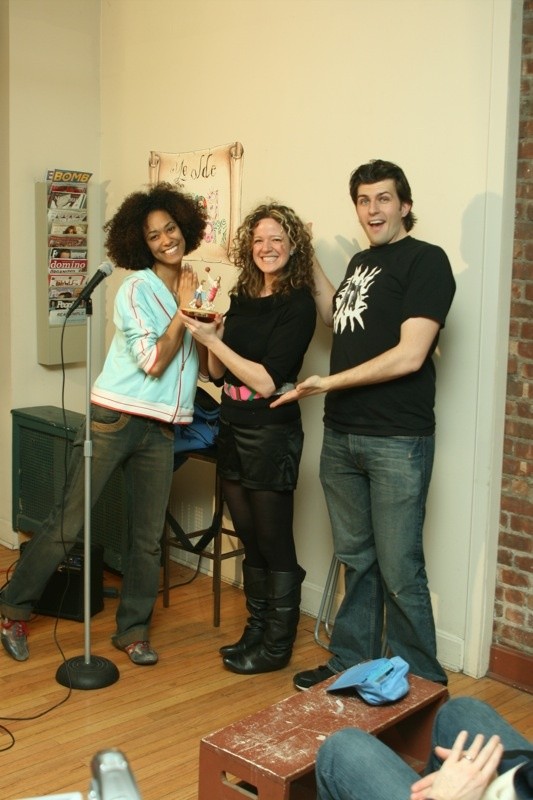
[45,169,92,327]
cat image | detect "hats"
[327,656,410,704]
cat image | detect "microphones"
[67,262,113,317]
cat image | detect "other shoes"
[0,614,29,661]
[126,641,158,664]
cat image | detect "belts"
[223,380,294,402]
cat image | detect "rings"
[463,753,475,762]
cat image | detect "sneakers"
[293,663,333,691]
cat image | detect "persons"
[0,180,212,666]
[316,697,533,800]
[270,160,457,692]
[177,206,317,674]
[189,272,221,310]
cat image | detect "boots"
[219,567,271,657]
[223,570,303,673]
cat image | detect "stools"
[161,445,245,627]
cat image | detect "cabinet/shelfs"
[198,660,448,800]
[9,406,125,573]
[35,181,88,366]
